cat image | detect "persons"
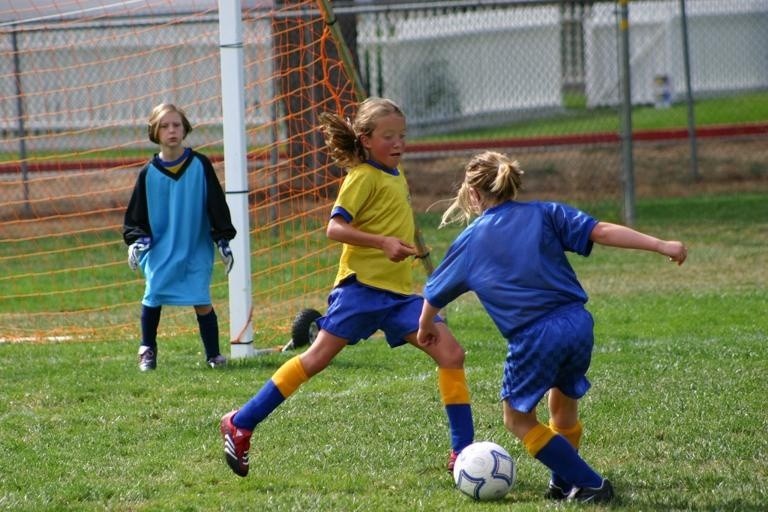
[217,94,475,478]
[123,101,237,371]
[417,151,689,501]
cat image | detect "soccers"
[454,441,514,502]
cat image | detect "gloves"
[218,237,234,275]
[128,243,144,270]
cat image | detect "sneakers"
[137,345,158,371]
[446,449,613,508]
[207,355,226,367]
[220,410,252,476]
